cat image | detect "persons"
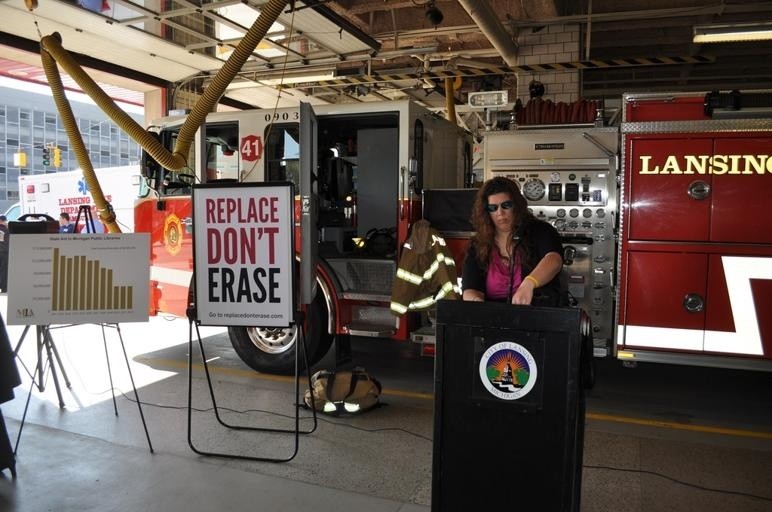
[0,214,8,292]
[80,209,104,234]
[58,212,74,234]
[461,176,563,307]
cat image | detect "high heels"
[0,454,17,478]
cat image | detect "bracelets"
[525,276,538,289]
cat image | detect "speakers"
[8,212,60,234]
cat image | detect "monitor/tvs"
[422,187,480,239]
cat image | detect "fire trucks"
[131,88,771,377]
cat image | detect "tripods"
[14,322,69,409]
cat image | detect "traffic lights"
[42,148,50,166]
[54,149,62,168]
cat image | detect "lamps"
[692,22,772,43]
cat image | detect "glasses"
[487,201,514,212]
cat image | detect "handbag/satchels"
[302,370,382,415]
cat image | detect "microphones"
[509,233,521,252]
